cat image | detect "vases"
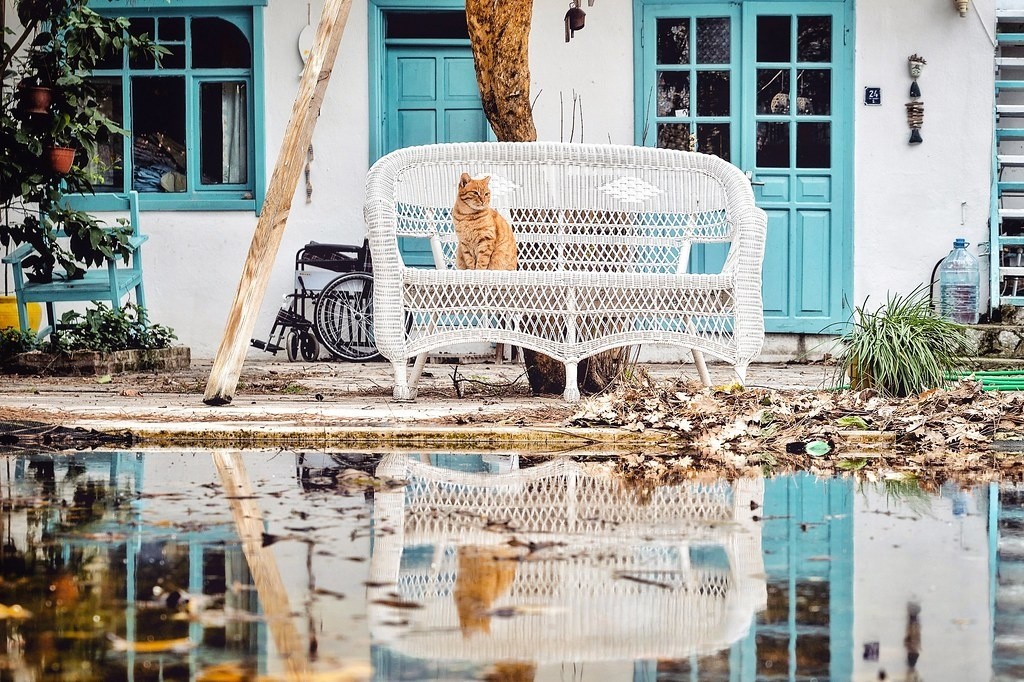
[48,145,77,173]
[17,84,55,116]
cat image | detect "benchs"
[370,453,767,666]
[367,141,769,401]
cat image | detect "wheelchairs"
[251,238,413,362]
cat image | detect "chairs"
[2,191,149,351]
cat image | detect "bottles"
[941,239,979,324]
[952,494,967,517]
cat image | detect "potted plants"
[797,290,980,396]
[0,201,43,340]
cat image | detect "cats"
[452,173,519,272]
[452,544,519,636]
